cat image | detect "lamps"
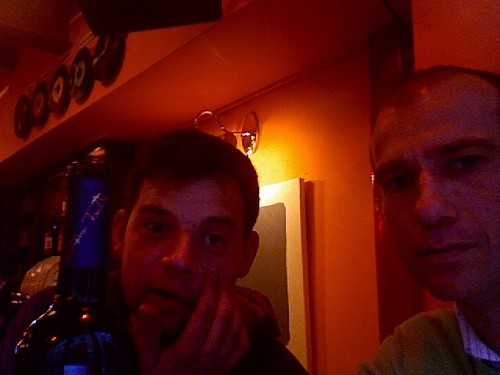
[195,109,258,154]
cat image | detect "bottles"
[41,206,60,256]
[57,200,67,255]
[16,157,138,375]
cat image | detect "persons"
[103,127,313,375]
[355,64,500,375]
